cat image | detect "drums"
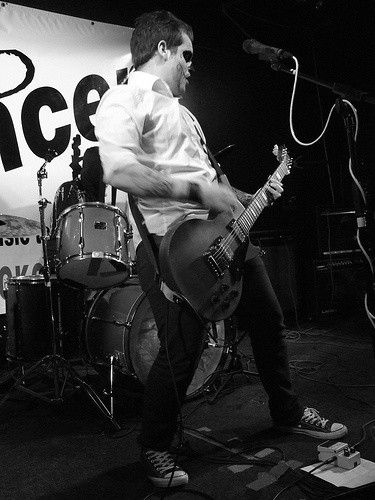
[52,202,132,290]
[82,275,226,401]
[6,275,55,365]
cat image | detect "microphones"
[242,39,293,63]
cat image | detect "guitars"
[157,142,293,322]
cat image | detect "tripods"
[1,149,122,431]
[202,143,260,404]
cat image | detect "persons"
[92,6,348,488]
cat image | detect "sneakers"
[273,408,348,439]
[140,448,188,487]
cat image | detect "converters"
[317,439,361,469]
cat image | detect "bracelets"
[189,181,202,202]
